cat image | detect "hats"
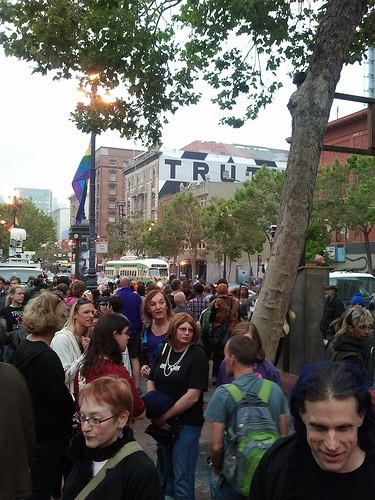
[351,293,364,307]
[324,285,338,292]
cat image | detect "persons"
[314,255,375,455]
[0,254,296,396]
[60,373,166,500]
[201,335,290,500]
[249,360,375,500]
[74,312,145,439]
[0,317,39,500]
[11,289,78,500]
[145,311,210,500]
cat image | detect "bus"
[103,255,171,283]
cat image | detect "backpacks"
[221,378,281,497]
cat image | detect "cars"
[327,270,375,311]
[0,264,45,286]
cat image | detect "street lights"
[83,66,100,313]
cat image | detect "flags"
[72,155,96,225]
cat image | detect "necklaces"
[164,343,190,376]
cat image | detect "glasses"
[115,329,131,337]
[75,411,122,427]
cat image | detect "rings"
[160,424,163,426]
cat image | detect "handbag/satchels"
[211,324,227,345]
[145,414,183,447]
